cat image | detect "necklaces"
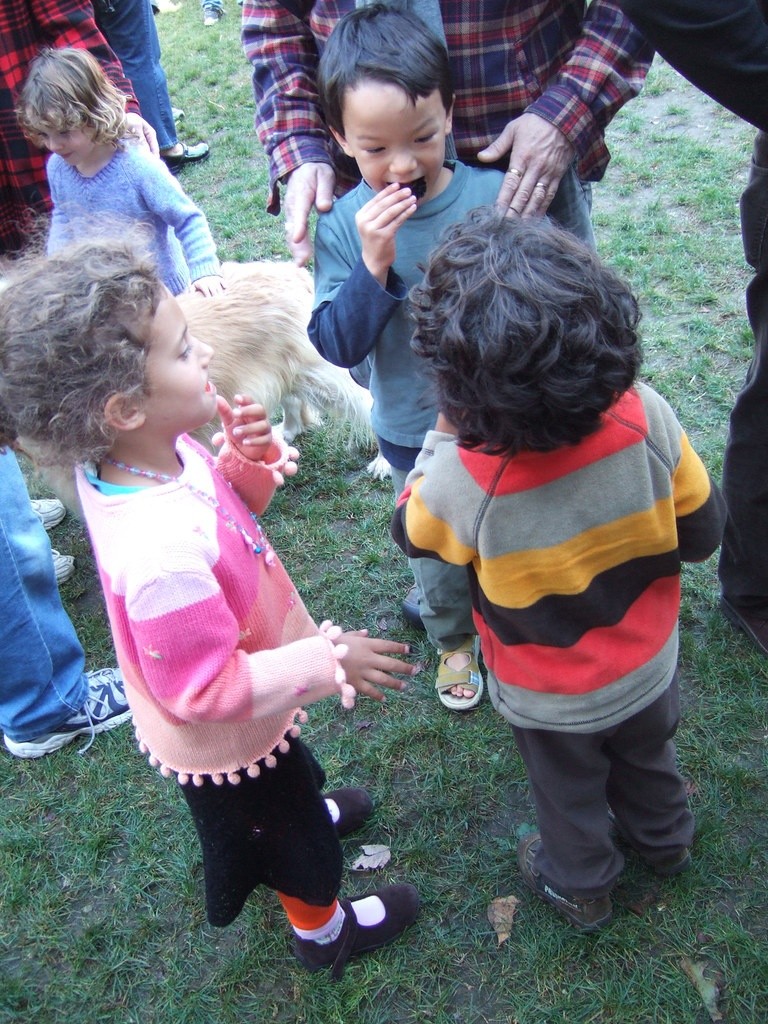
[98,436,275,569]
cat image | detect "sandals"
[435,633,484,710]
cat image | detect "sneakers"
[3,667,132,759]
[31,498,66,530]
[51,549,77,585]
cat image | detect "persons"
[307,3,561,710]
[0,218,421,987]
[0,436,132,760]
[0,0,227,305]
[385,209,729,930]
[240,0,657,623]
[613,0,768,654]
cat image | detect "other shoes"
[294,884,418,981]
[172,107,187,121]
[720,598,768,654]
[323,786,373,837]
[204,10,218,26]
[160,143,210,174]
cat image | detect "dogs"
[13,256,393,523]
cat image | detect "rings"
[507,168,523,178]
[536,183,548,190]
